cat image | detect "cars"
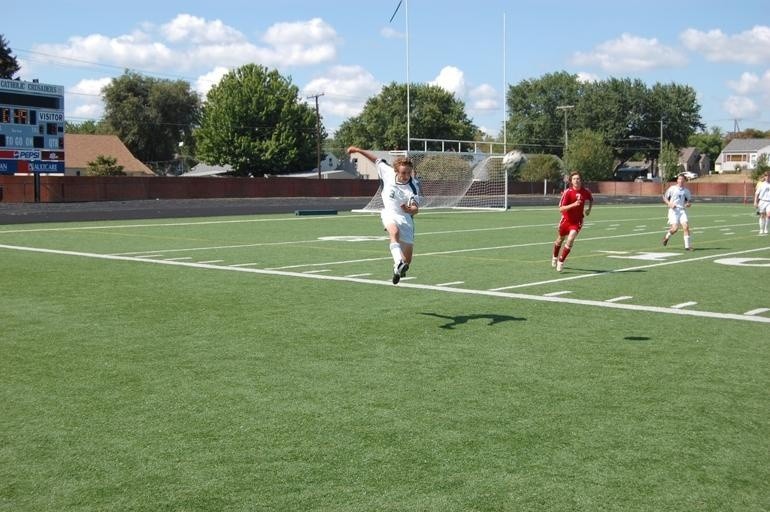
[634,176,647,182]
[674,171,698,180]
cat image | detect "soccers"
[503,152,526,175]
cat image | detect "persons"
[552,172,593,271]
[662,174,695,251]
[348,146,420,284]
[753,171,770,235]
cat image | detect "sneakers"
[557,258,564,271]
[662,236,668,246]
[399,263,409,277]
[393,264,400,284]
[552,254,558,267]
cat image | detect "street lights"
[629,135,663,181]
